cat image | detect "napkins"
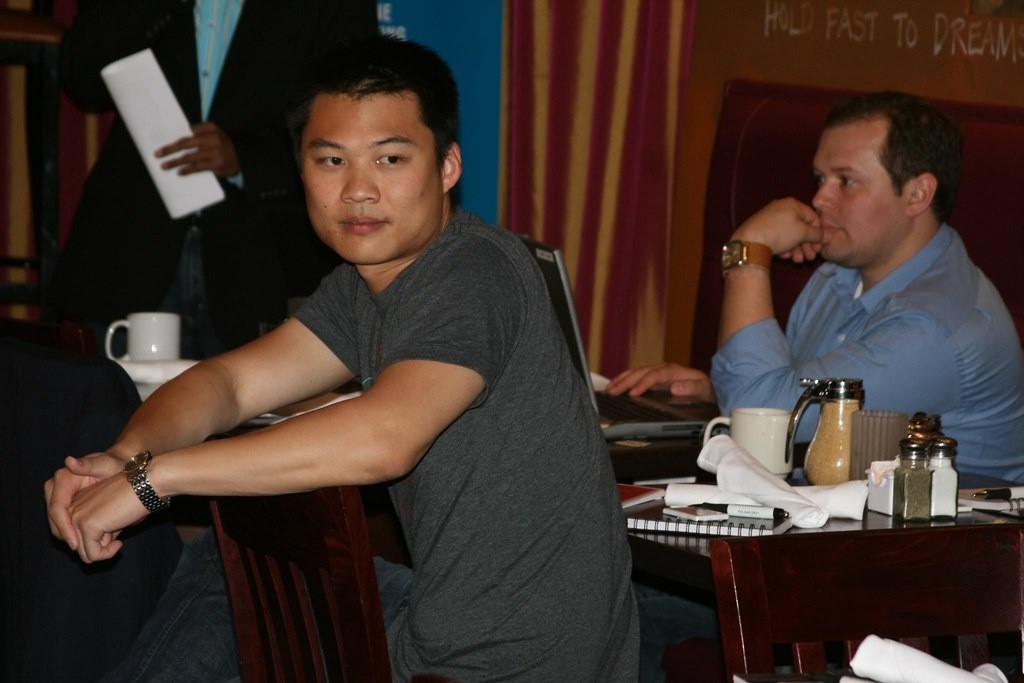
[839,635,991,683]
[662,434,869,529]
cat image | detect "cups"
[849,409,911,481]
[105,311,181,362]
[703,408,795,481]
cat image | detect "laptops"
[514,231,734,439]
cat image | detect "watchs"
[719,240,773,278]
[123,450,172,515]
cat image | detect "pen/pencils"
[972,486,1024,501]
[689,502,785,523]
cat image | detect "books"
[958,487,1024,512]
[627,504,793,537]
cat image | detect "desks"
[623,473,1024,683]
[598,387,722,481]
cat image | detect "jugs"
[784,377,864,484]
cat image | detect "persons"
[54,0,380,349]
[43,31,642,683]
[605,91,1024,486]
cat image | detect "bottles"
[894,438,931,521]
[924,436,960,520]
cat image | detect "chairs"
[201,429,394,683]
[707,522,1024,683]
